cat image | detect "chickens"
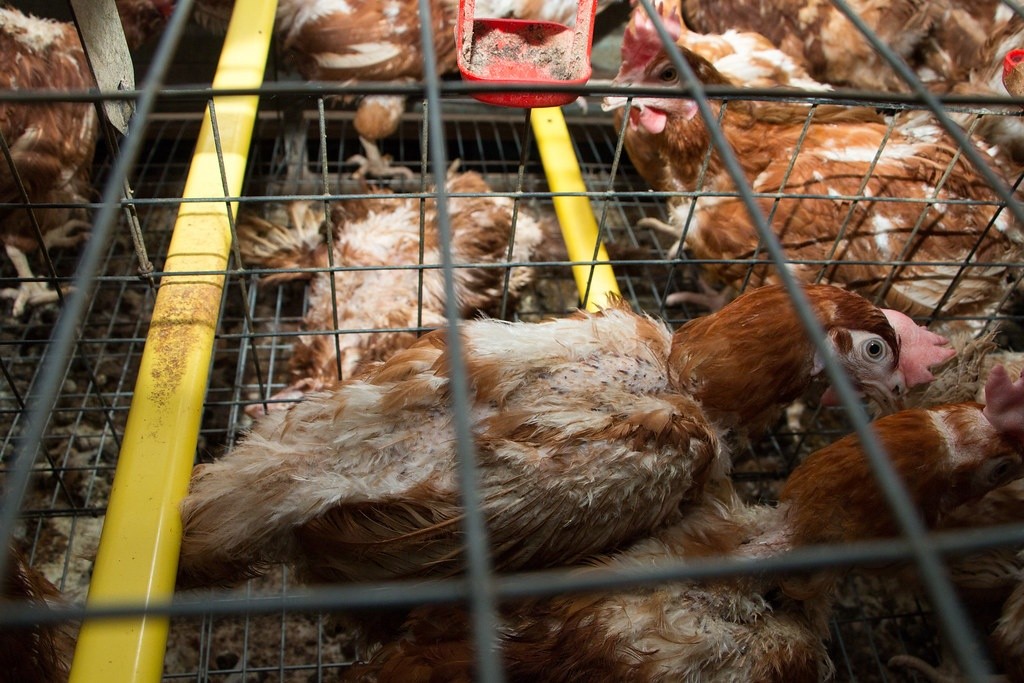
[1,1,1024,682]
